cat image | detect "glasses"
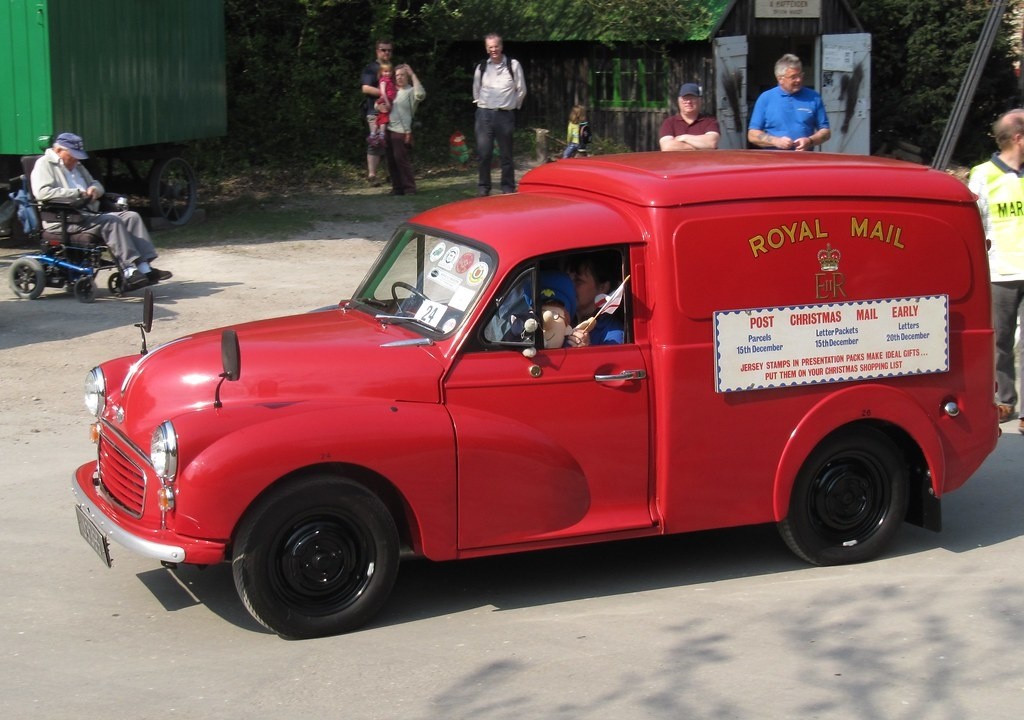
[782,72,805,81]
[378,48,393,53]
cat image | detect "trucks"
[1,0,229,224]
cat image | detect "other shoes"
[997,403,1015,422]
[123,270,149,290]
[379,137,388,148]
[1018,420,1024,433]
[389,189,399,195]
[367,137,378,147]
[145,267,172,281]
[366,176,380,187]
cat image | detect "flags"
[595,284,624,313]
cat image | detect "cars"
[73,149,1001,640]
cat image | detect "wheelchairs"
[9,156,160,304]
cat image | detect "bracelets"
[808,137,813,146]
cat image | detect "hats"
[57,133,89,160]
[679,83,699,97]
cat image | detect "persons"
[968,109,1024,433]
[659,83,721,152]
[565,246,624,347]
[30,133,172,288]
[748,54,831,151]
[563,105,586,158]
[360,40,425,195]
[540,300,573,348]
[473,33,526,196]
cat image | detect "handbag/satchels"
[404,132,415,148]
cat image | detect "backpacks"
[578,121,592,145]
